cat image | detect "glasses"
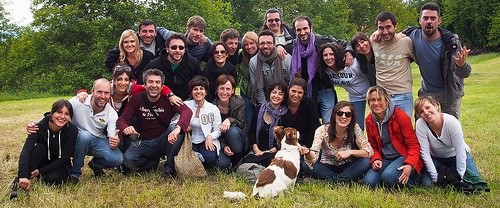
[259,42,274,45]
[336,111,352,117]
[268,18,280,23]
[213,50,227,54]
[113,65,131,74]
[169,45,185,50]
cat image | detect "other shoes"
[88,161,107,177]
[70,176,78,185]
[165,172,174,177]
[154,158,160,171]
[9,182,21,201]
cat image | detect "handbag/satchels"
[174,132,207,180]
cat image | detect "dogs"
[251,124,302,201]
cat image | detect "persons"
[172,74,222,177]
[140,37,205,95]
[202,7,375,131]
[9,99,78,200]
[156,16,213,63]
[105,21,164,80]
[207,74,246,174]
[298,101,373,183]
[362,86,424,187]
[371,2,471,121]
[243,80,288,167]
[277,77,319,170]
[414,96,480,187]
[368,13,417,118]
[115,69,192,179]
[75,67,183,175]
[25,78,124,186]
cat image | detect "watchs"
[174,125,181,128]
[167,92,174,97]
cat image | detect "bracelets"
[305,148,310,155]
